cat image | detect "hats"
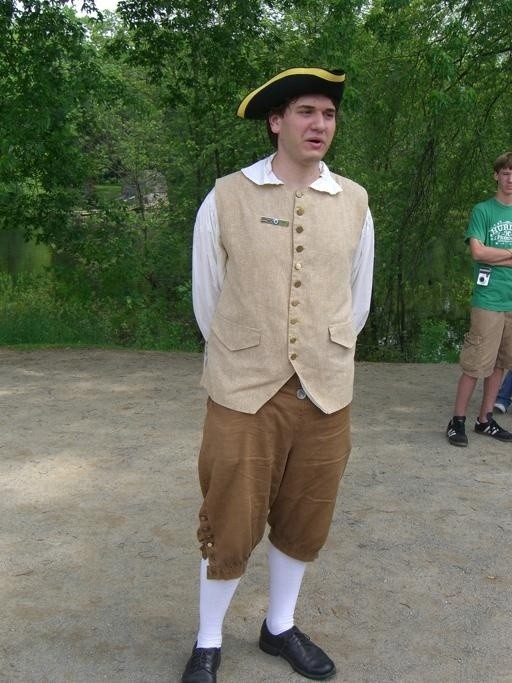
[237,69,346,118]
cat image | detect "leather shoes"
[258,617,334,679]
[181,639,219,683]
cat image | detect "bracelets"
[507,248,512,255]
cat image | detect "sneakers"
[447,416,468,446]
[493,403,506,414]
[475,412,511,440]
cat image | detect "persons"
[182,65,378,682]
[493,369,512,414]
[446,151,512,445]
